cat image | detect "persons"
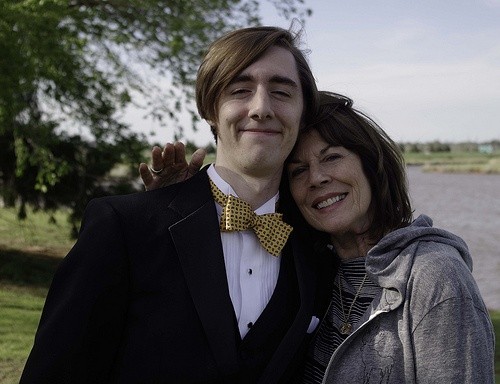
[139,91,495,384]
[20,27,338,384]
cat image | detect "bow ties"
[208,177,293,257]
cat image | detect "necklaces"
[338,271,367,334]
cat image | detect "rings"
[150,168,164,174]
